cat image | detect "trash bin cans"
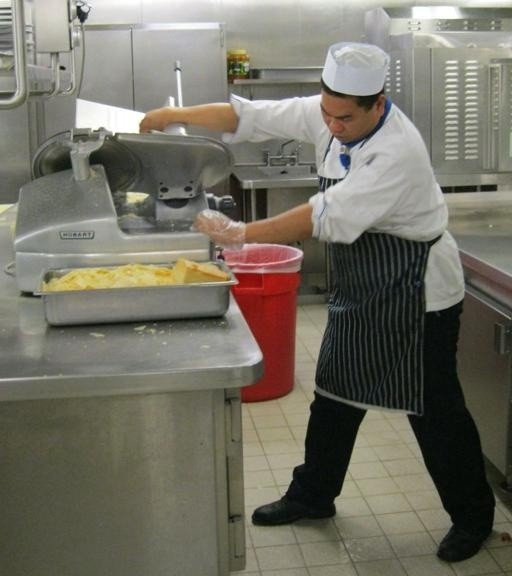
[215,241,304,402]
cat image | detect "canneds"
[227,49,250,83]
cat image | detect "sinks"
[258,164,316,178]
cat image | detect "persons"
[138,40,496,562]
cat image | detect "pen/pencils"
[365,154,378,166]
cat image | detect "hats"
[322,39,392,97]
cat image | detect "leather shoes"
[251,480,337,525]
[437,511,495,562]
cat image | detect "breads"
[43,258,230,292]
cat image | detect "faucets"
[281,139,302,154]
